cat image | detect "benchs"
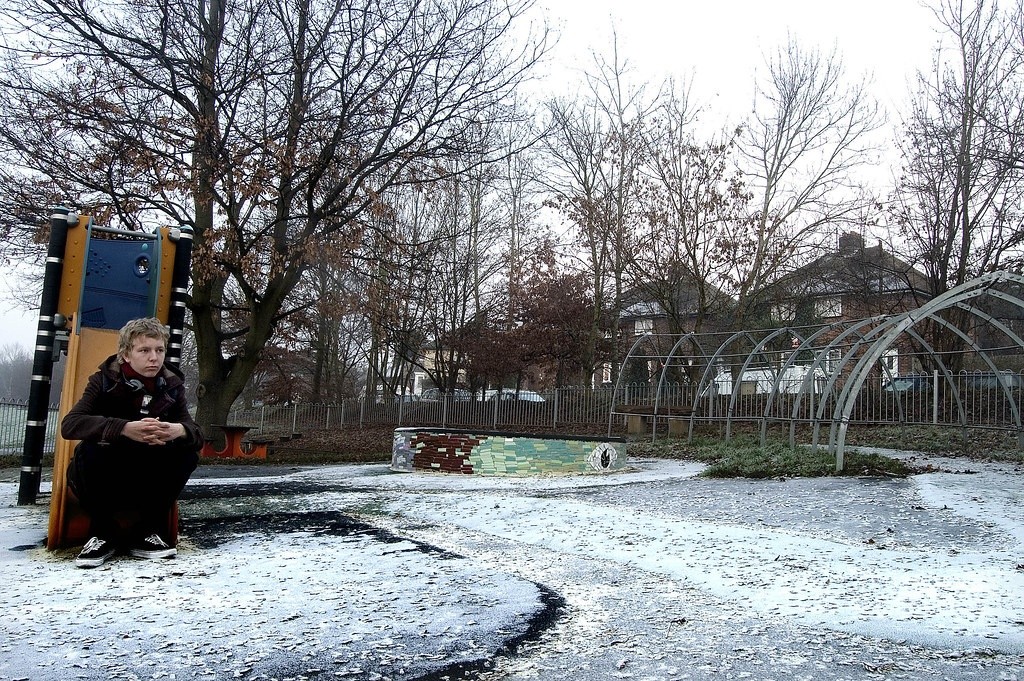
[613,403,701,439]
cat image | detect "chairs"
[203,437,268,460]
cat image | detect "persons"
[60,317,204,569]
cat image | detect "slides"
[47,313,181,552]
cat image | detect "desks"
[210,423,259,459]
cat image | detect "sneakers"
[76,537,115,566]
[130,533,177,559]
